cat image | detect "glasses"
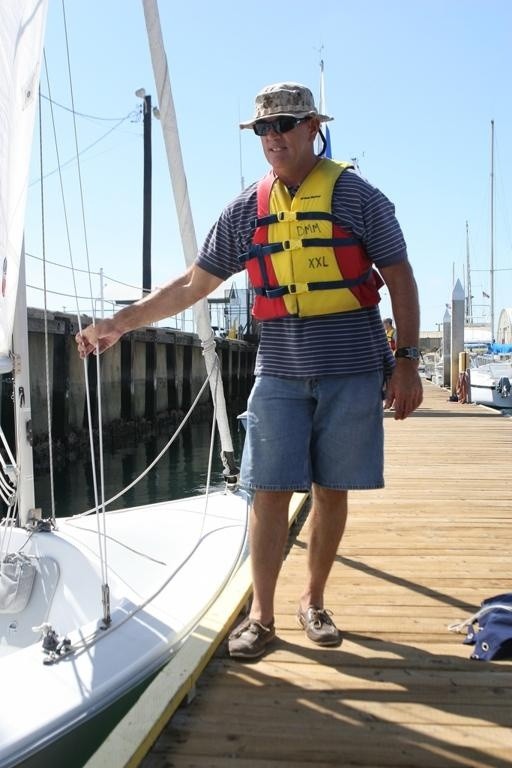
[253,116,312,136]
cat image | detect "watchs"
[395,347,420,361]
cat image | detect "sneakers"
[229,618,275,658]
[298,606,341,645]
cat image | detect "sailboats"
[0,0,251,767]
[419,119,511,409]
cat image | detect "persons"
[383,317,397,359]
[76,83,424,662]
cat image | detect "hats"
[239,82,334,130]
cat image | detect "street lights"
[135,87,161,297]
